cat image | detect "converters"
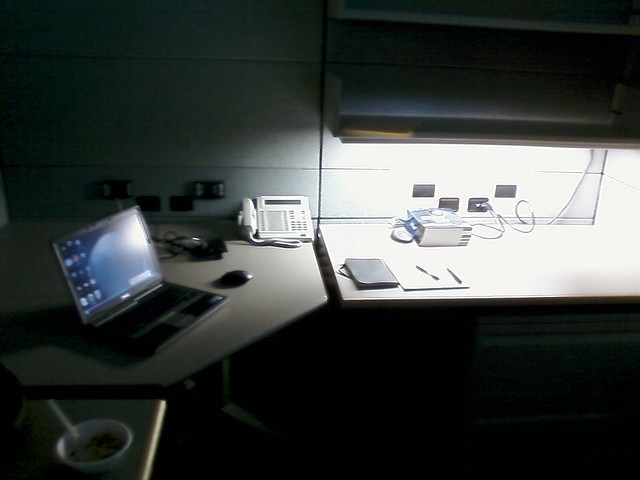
[189,247,222,259]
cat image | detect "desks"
[1,397,167,480]
[318,221,640,308]
[1,219,333,386]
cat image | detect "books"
[382,256,470,291]
[345,258,399,288]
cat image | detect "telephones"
[237,196,315,248]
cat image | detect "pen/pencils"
[416,265,438,281]
[447,268,461,283]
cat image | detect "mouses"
[220,270,255,286]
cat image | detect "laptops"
[49,205,229,354]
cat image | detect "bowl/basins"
[56,419,133,474]
